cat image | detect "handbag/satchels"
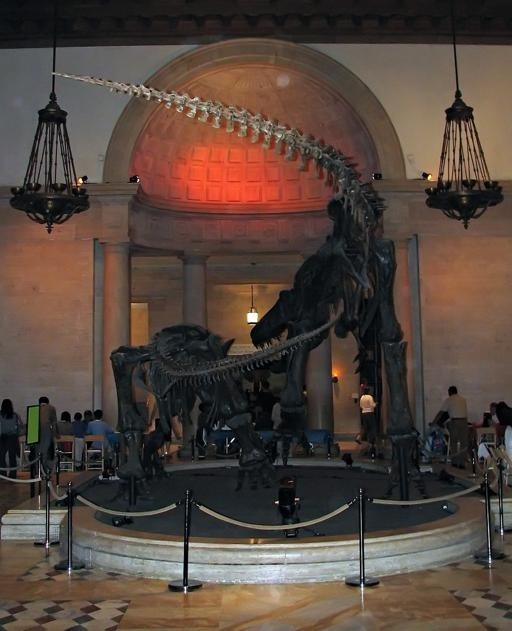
[15,423,27,436]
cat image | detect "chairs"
[16,435,105,478]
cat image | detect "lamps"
[246,285,259,325]
[424,2,505,230]
[9,47,90,235]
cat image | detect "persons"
[1,396,213,483]
[427,385,512,490]
[359,388,380,446]
[248,379,293,460]
